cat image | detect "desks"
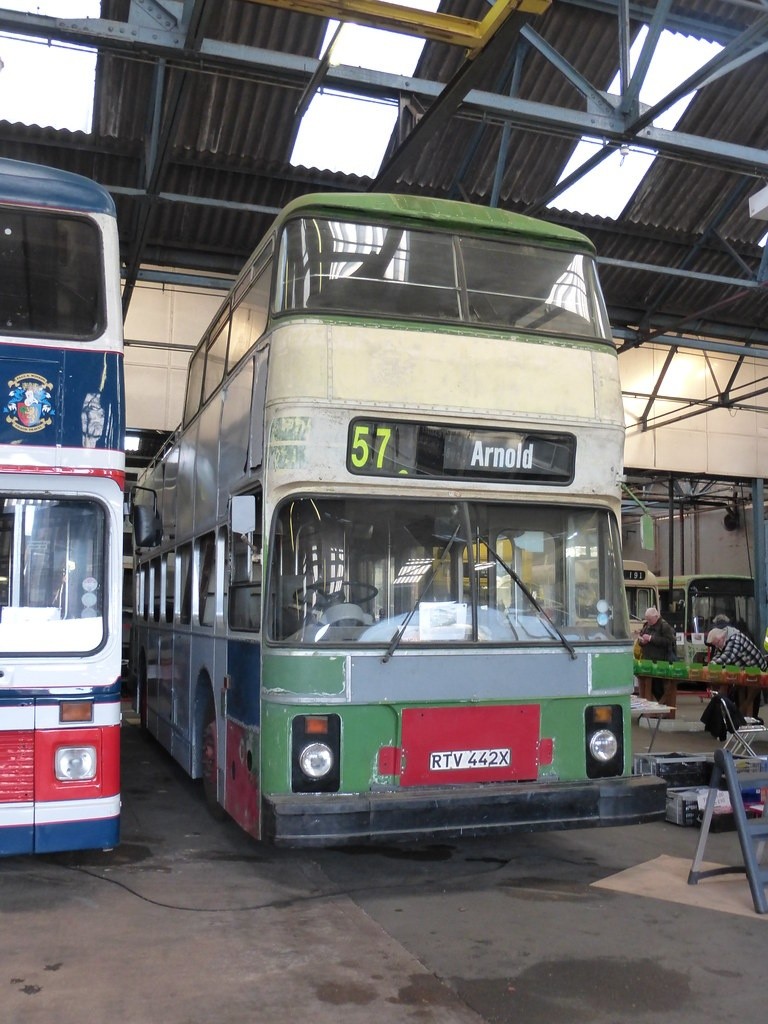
[630,697,672,754]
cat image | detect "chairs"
[711,691,767,757]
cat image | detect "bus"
[429,540,545,613]
[1,154,163,863]
[128,191,662,852]
[533,559,662,637]
[657,575,758,686]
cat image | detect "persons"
[638,607,677,702]
[704,614,768,717]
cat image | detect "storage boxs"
[634,752,768,833]
